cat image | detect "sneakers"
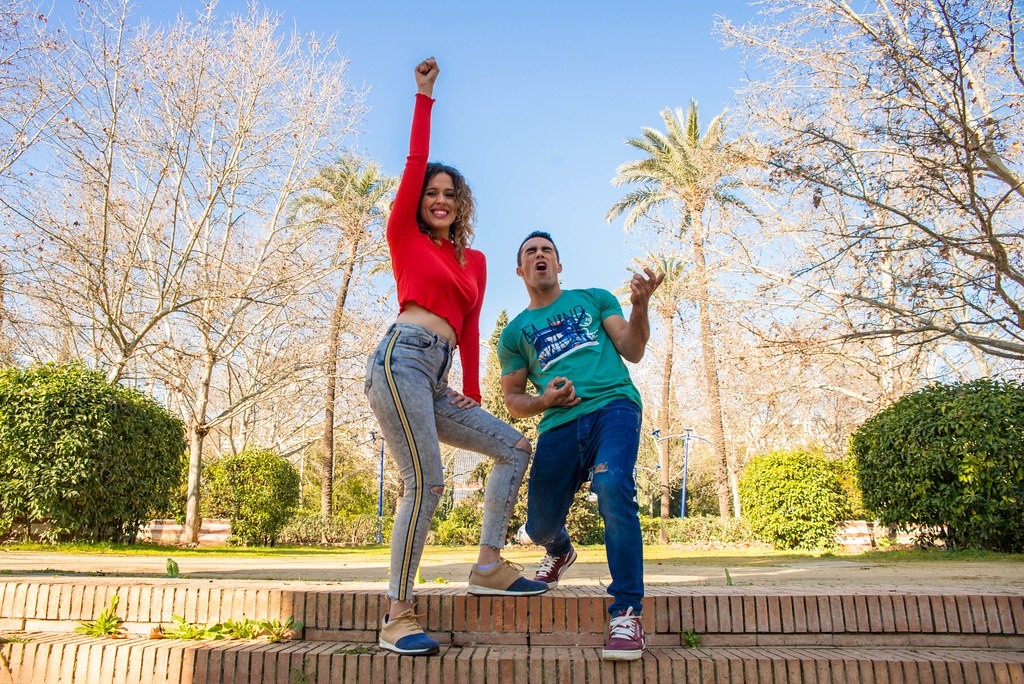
[601,605,647,661]
[468,556,549,596]
[534,546,578,590]
[378,603,440,656]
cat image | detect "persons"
[363,56,548,655]
[497,231,666,660]
